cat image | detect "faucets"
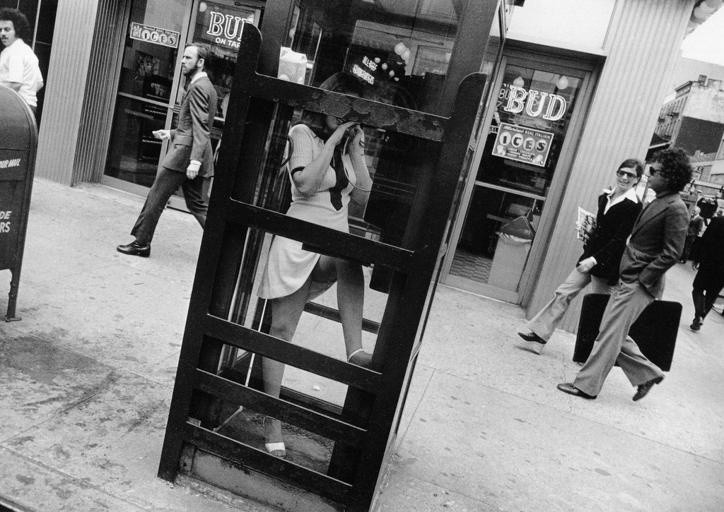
[263,415,286,459]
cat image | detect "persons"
[678,206,723,330]
[0,8,44,112]
[117,43,219,257]
[258,71,375,459]
[557,148,693,401]
[517,159,644,355]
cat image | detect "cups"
[616,169,637,180]
[650,167,660,175]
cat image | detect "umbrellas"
[633,373,665,401]
[117,239,150,257]
[558,383,597,399]
[690,315,704,330]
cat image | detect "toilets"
[342,124,358,144]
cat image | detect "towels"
[487,216,535,292]
[0,86,38,322]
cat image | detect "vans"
[514,332,546,355]
